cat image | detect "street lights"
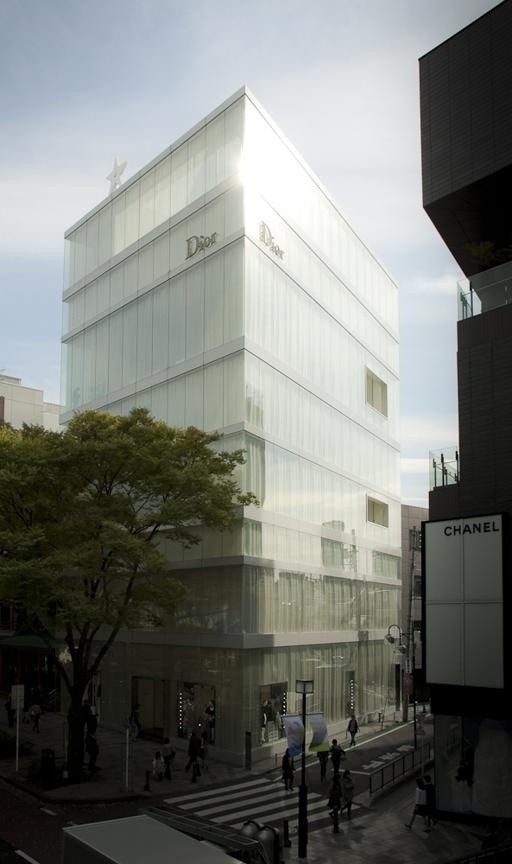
[385,623,406,711]
[295,677,315,859]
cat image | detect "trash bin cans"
[240,820,285,864]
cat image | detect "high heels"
[403,820,440,833]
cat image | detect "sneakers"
[329,811,352,820]
[282,786,295,792]
[347,741,360,747]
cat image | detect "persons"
[4,697,16,727]
[29,703,42,733]
[162,738,175,780]
[153,751,165,781]
[187,732,201,767]
[205,704,215,741]
[424,775,437,825]
[282,748,296,791]
[85,730,99,773]
[130,704,141,741]
[347,715,360,746]
[405,779,431,832]
[261,700,268,742]
[317,739,354,817]
[200,744,208,768]
